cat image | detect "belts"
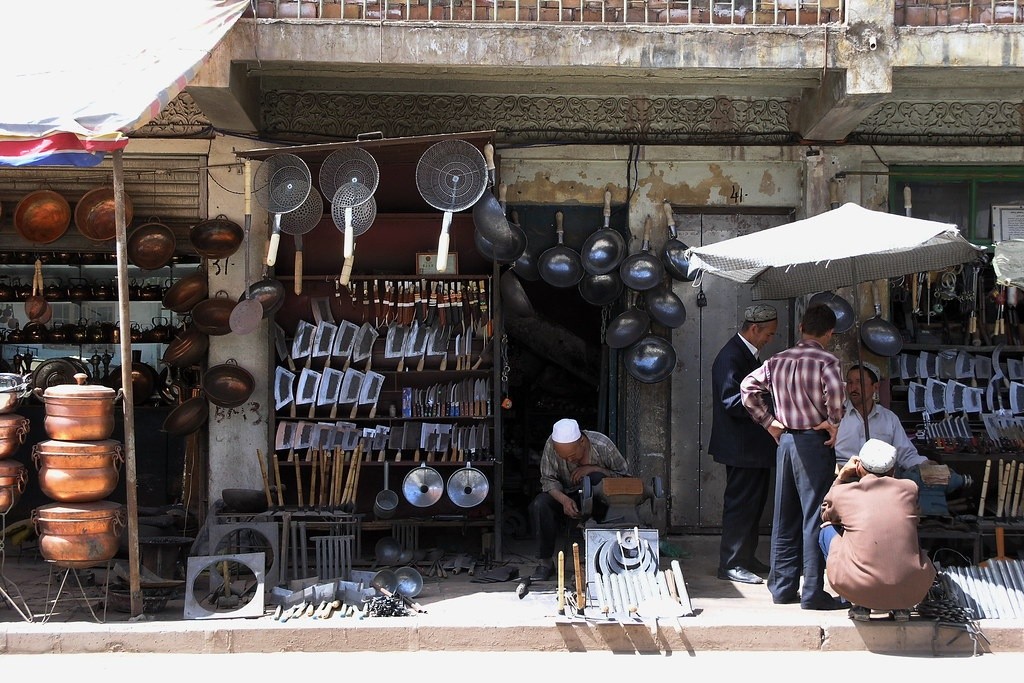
[784,428,828,435]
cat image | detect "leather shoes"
[718,566,763,583]
[749,560,769,572]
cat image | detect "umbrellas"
[682,201,991,442]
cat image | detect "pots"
[109,363,159,408]
[810,291,855,334]
[14,179,72,250]
[124,216,177,269]
[75,174,134,247]
[222,482,287,513]
[159,265,285,440]
[0,367,124,571]
[472,142,699,382]
[190,215,243,259]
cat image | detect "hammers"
[218,560,239,607]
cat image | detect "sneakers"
[531,560,555,580]
[888,609,910,622]
[800,597,844,609]
[774,595,801,603]
[847,605,871,622]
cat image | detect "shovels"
[272,577,376,623]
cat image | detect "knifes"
[896,350,1023,457]
[273,277,494,465]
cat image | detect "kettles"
[0,250,182,344]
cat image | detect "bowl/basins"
[861,281,902,356]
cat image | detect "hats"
[850,360,880,382]
[859,438,898,474]
[551,418,581,443]
[744,303,777,323]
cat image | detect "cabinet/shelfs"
[887,339,1024,566]
[263,209,503,570]
[0,262,205,343]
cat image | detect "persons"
[831,358,940,483]
[738,298,853,611]
[706,303,779,586]
[818,437,938,623]
[528,417,631,581]
